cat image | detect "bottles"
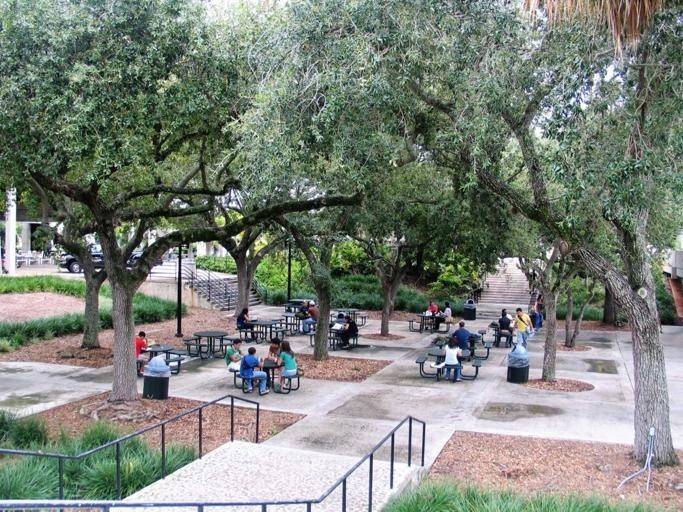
[309,300,316,306]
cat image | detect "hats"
[55,244,162,275]
[0,247,25,273]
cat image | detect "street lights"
[526,325,535,336]
[241,359,252,370]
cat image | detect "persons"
[239,347,270,395]
[427,293,545,382]
[340,316,358,349]
[236,308,255,340]
[134,331,147,376]
[225,338,244,372]
[299,301,308,314]
[301,300,319,333]
[273,341,298,393]
[267,337,282,385]
[332,314,347,326]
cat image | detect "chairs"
[444,374,450,380]
[141,367,146,375]
[259,389,270,396]
[244,386,254,393]
[455,378,462,382]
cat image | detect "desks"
[281,238,297,314]
[172,242,191,337]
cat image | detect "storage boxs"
[136,295,372,396]
[406,308,531,384]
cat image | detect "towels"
[464,299,476,320]
[143,357,171,400]
[507,344,529,383]
